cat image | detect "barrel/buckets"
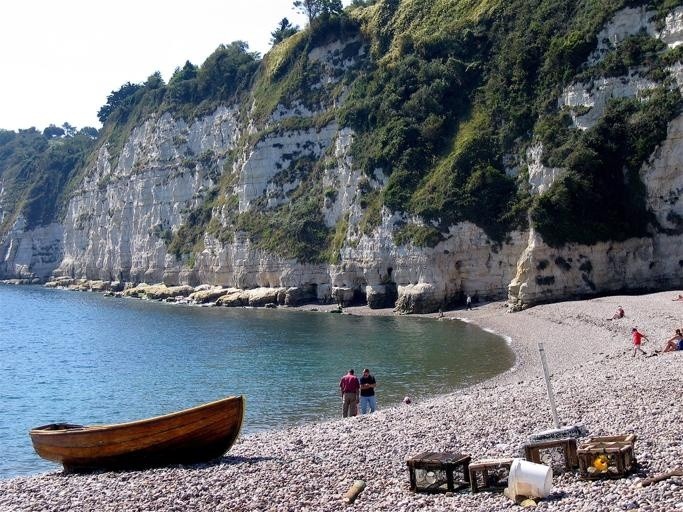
[508,457,553,500]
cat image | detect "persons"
[605,305,624,320]
[631,328,648,358]
[663,328,682,351]
[465,293,471,310]
[339,368,359,418]
[358,368,377,415]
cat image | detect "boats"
[26,392,248,469]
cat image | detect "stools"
[468,459,515,493]
[584,432,635,442]
[406,452,471,493]
[577,442,634,482]
[523,435,578,474]
